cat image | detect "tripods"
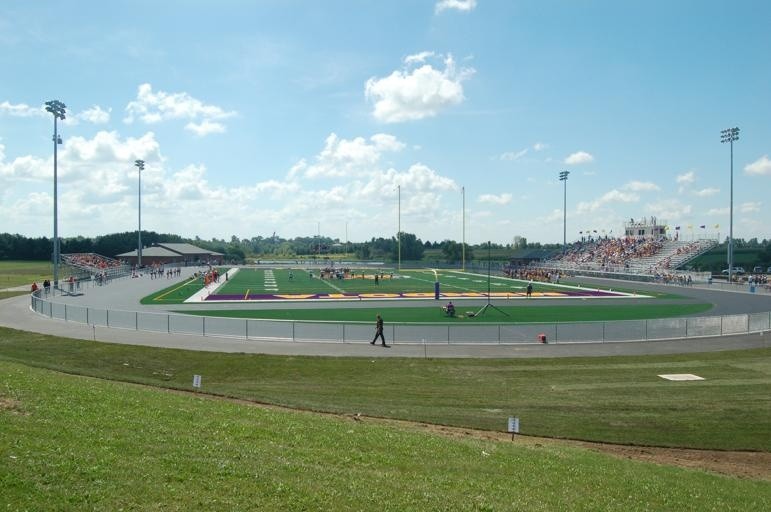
[473,241,511,319]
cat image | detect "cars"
[721,266,746,275]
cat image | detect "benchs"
[532,236,714,273]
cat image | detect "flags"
[664,224,670,231]
[687,224,694,230]
[699,224,707,229]
[675,226,681,230]
[713,223,720,230]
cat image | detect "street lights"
[720,126,740,276]
[558,170,570,244]
[45,99,65,291]
[135,159,145,265]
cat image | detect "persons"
[42,280,48,294]
[370,313,387,347]
[225,271,228,281]
[732,271,769,288]
[526,282,532,297]
[46,279,49,294]
[501,230,700,288]
[308,267,364,281]
[63,254,221,291]
[379,269,383,278]
[446,301,455,317]
[374,272,378,285]
[389,271,393,280]
[31,282,37,291]
[288,271,292,281]
